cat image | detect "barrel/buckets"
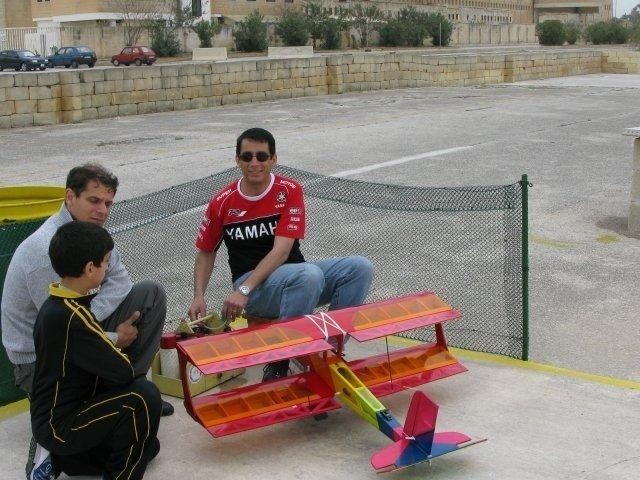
[159,334,183,381]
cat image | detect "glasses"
[236,152,272,162]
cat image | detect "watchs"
[239,285,252,297]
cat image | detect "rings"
[231,309,237,313]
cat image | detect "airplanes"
[175,291,489,475]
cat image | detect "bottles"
[159,331,180,381]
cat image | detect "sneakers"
[25,434,56,480]
[261,357,291,384]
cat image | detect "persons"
[22,219,162,480]
[187,127,376,385]
[0,162,175,418]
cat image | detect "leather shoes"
[160,397,174,416]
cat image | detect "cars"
[0,49,48,72]
[45,46,97,69]
[110,45,156,66]
